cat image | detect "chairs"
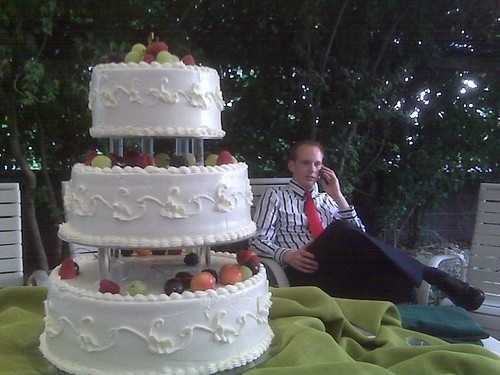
[242,178,299,289]
[418,183,500,338]
[0,182,24,289]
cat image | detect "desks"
[0,286,500,375]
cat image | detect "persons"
[249,140,486,312]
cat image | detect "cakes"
[57,147,257,248]
[37,248,274,375]
[88,42,227,138]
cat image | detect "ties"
[304,191,325,240]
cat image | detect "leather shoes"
[440,274,485,311]
